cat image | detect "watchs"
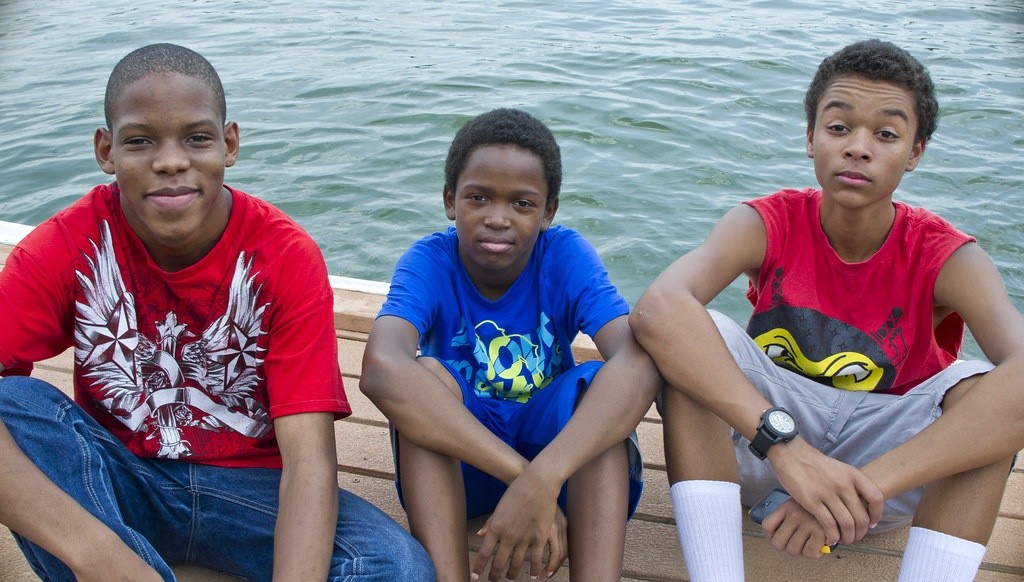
[748,406,801,462]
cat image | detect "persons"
[358,108,661,582]
[629,39,1024,582]
[0,43,436,582]
